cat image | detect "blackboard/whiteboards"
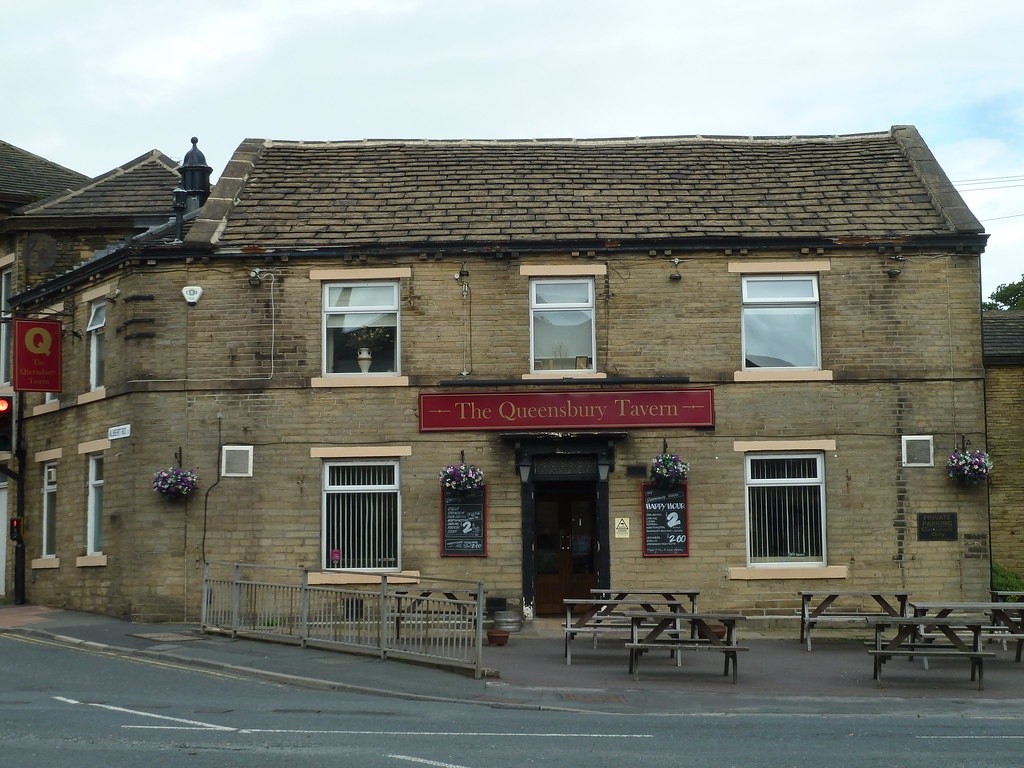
[640,481,690,558]
[438,483,489,558]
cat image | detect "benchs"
[386,586,1024,693]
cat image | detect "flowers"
[152,467,199,495]
[652,453,690,486]
[438,462,487,494]
[946,449,994,481]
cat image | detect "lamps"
[888,255,905,275]
[249,272,262,287]
[454,269,469,280]
[63,297,74,311]
[669,257,682,280]
